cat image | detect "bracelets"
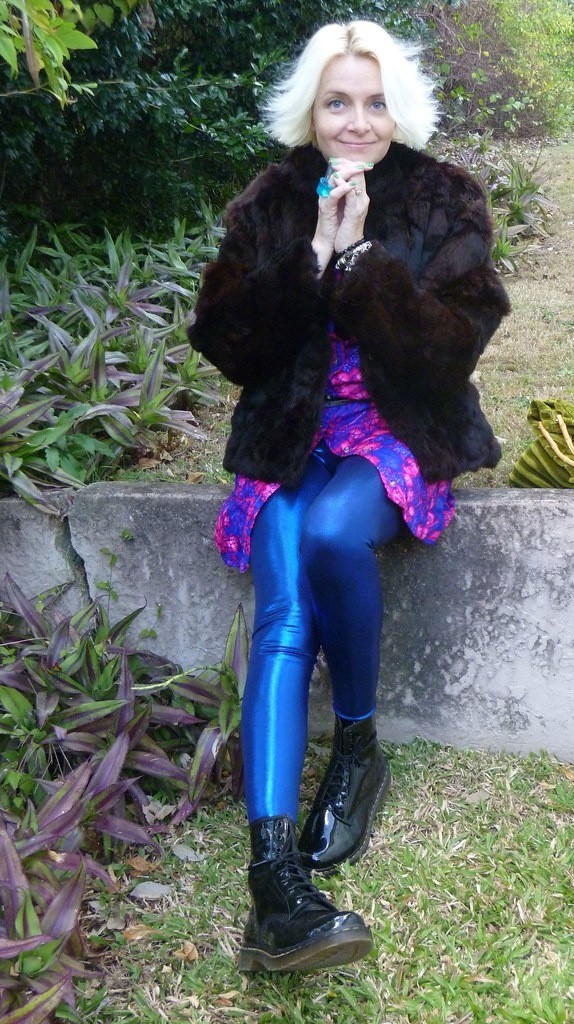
[335,240,374,273]
[334,235,365,261]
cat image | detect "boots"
[233,818,371,974]
[297,718,393,877]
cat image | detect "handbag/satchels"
[510,401,574,488]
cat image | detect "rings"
[315,176,336,199]
[353,189,365,196]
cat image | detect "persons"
[185,20,514,976]
